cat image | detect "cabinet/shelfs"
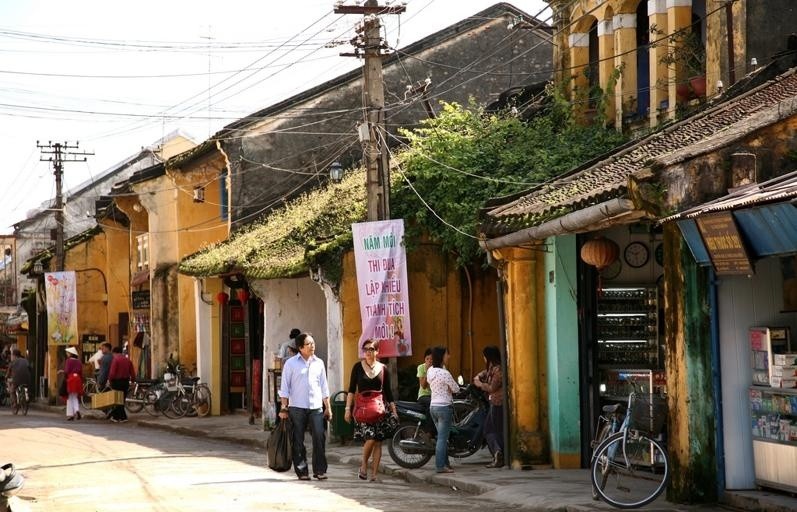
[596,365,670,472]
[593,279,659,373]
[746,383,796,496]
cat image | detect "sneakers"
[436,466,455,473]
[67,416,74,422]
[110,416,119,423]
[358,465,368,481]
[76,411,82,420]
[119,418,129,424]
[494,450,504,468]
[369,477,383,484]
[485,460,500,468]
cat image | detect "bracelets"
[280,404,289,412]
[344,406,351,410]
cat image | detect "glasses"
[363,347,375,352]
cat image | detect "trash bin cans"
[330,390,354,436]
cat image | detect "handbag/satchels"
[353,389,386,424]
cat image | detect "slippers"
[313,474,328,480]
[298,475,311,481]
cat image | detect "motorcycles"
[389,374,497,471]
[0,461,25,512]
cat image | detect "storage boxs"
[768,353,796,389]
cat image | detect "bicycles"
[587,373,668,507]
[122,353,210,423]
[81,376,116,411]
[0,373,32,419]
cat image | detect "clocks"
[655,274,665,300]
[654,239,663,268]
[596,259,622,281]
[621,241,649,269]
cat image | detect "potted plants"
[569,66,603,127]
[640,21,709,100]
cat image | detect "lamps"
[131,200,145,212]
[190,169,207,205]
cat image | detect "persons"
[416,347,447,444]
[278,328,300,358]
[88,347,104,380]
[281,339,298,365]
[108,347,135,422]
[56,346,83,421]
[97,342,113,385]
[426,344,455,474]
[343,339,398,480]
[4,349,31,408]
[472,345,503,467]
[278,332,333,480]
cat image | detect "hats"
[64,346,80,356]
